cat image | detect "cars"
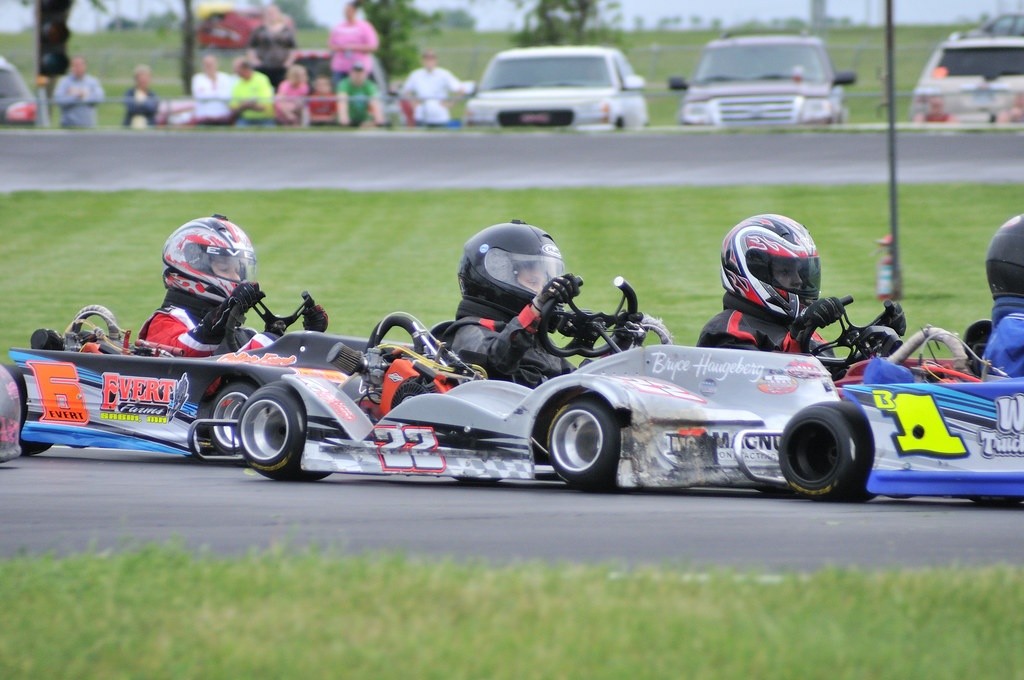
[978,14,1024,35]
[198,10,293,49]
[460,44,648,130]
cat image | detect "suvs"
[0,58,38,126]
[668,29,858,128]
[911,32,1024,125]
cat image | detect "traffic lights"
[37,0,71,75]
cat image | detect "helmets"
[720,214,821,318]
[458,219,565,314]
[986,213,1024,300]
[161,214,256,305]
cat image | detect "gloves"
[883,303,906,337]
[790,296,845,343]
[614,309,644,342]
[532,273,580,312]
[202,282,266,343]
[302,304,329,332]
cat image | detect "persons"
[983,214,1024,377]
[443,219,649,390]
[135,213,328,358]
[914,91,1024,124]
[53,2,462,127]
[696,213,902,383]
